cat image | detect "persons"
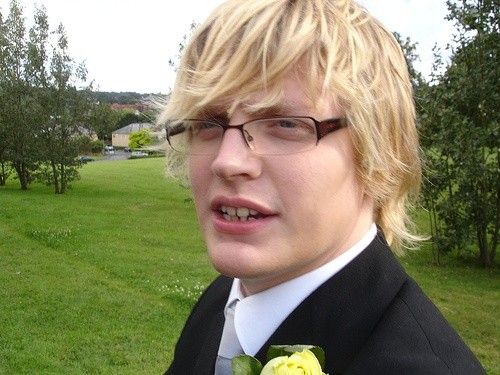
[155,0,489,375]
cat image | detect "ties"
[214,302,244,375]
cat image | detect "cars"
[74,157,95,163]
[128,151,149,158]
[105,145,115,154]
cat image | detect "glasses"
[165,116,348,155]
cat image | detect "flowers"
[230,344,329,375]
